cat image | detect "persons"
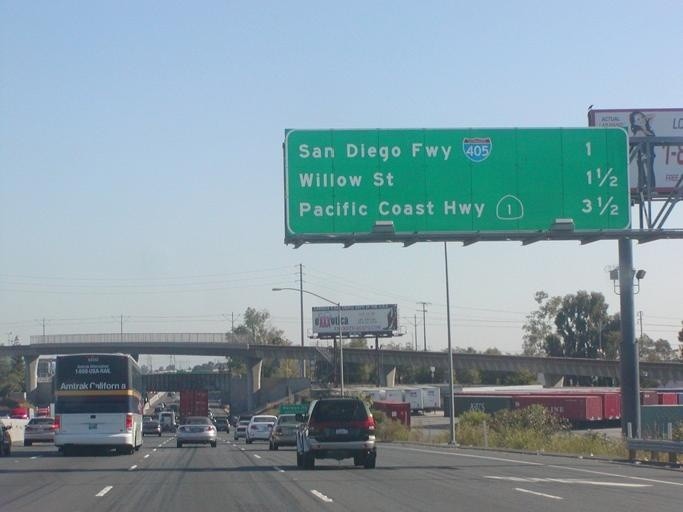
[627,110,656,188]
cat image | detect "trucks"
[179,389,209,418]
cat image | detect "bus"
[52,353,149,455]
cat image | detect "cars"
[0,403,56,456]
[176,416,218,448]
[268,414,307,451]
[208,407,253,440]
[143,391,180,436]
[245,414,278,444]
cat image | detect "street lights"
[272,287,344,398]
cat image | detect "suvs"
[295,395,377,469]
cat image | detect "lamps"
[552,218,575,232]
[373,221,394,233]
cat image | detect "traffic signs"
[280,126,632,243]
